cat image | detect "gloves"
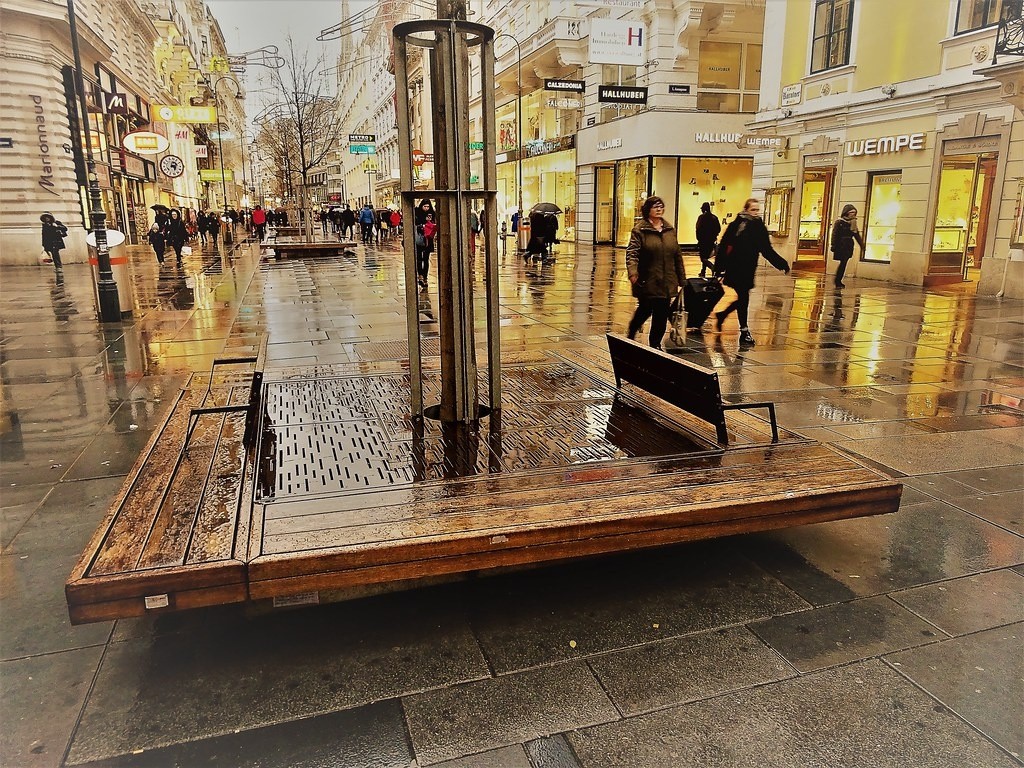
[782,265,791,275]
[830,247,834,252]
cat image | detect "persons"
[413,198,437,284]
[831,205,866,288]
[696,202,721,276]
[625,196,686,351]
[500,119,517,152]
[39,212,68,273]
[471,209,560,265]
[146,204,403,265]
[712,199,792,345]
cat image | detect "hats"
[171,210,177,214]
[153,223,160,230]
[846,209,856,213]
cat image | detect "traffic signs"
[349,134,376,143]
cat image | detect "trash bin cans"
[517,216,531,252]
[86,229,134,316]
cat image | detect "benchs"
[68,333,903,624]
[268,221,320,234]
[260,229,357,260]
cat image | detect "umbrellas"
[150,205,168,212]
[533,203,561,212]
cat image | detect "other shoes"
[698,273,705,278]
[419,279,425,287]
[739,330,756,344]
[161,262,164,267]
[55,267,62,272]
[542,260,547,264]
[522,254,529,264]
[158,263,161,267]
[712,270,715,277]
[715,310,725,332]
[424,281,428,286]
[835,281,846,288]
[651,344,662,352]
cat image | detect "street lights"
[241,129,258,231]
[214,76,245,243]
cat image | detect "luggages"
[668,270,724,329]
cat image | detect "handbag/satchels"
[371,223,379,237]
[670,288,689,345]
[415,225,424,247]
[380,220,387,230]
[423,220,437,239]
[181,242,193,255]
[54,219,67,237]
